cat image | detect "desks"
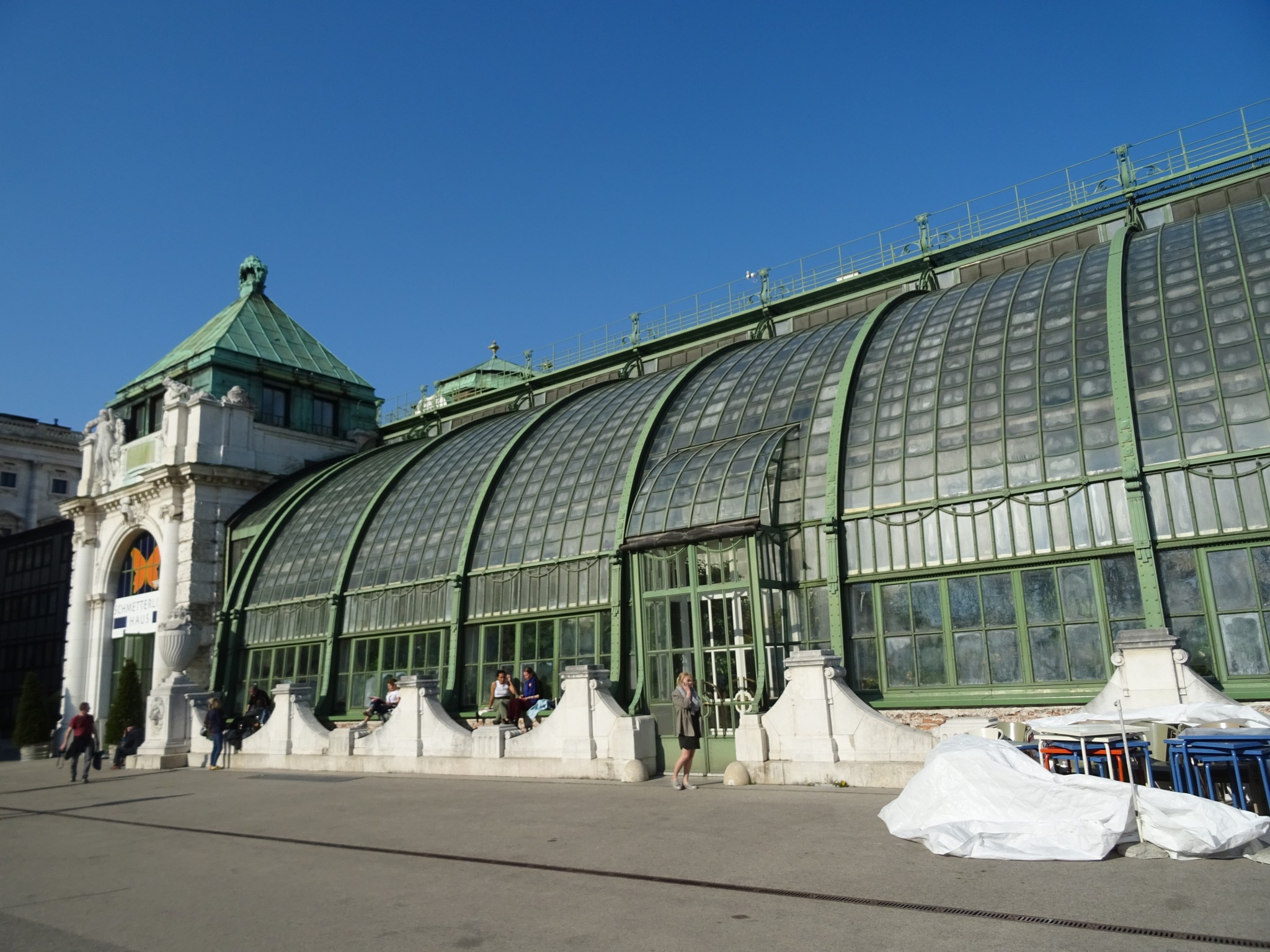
[1005,723,1270,816]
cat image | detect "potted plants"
[104,658,145,763]
[12,661,57,761]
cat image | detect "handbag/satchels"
[199,728,207,737]
[93,747,101,770]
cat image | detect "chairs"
[963,720,1034,758]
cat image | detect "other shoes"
[359,721,367,725]
[507,720,514,724]
[70,777,75,782]
[682,782,697,789]
[110,764,120,770]
[493,719,497,725]
[210,765,223,769]
[83,779,87,783]
[671,779,682,790]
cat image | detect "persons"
[162,377,212,404]
[60,702,99,784]
[485,670,517,725]
[204,697,225,770]
[501,666,543,724]
[91,408,126,487]
[358,678,400,727]
[672,672,702,790]
[111,723,141,770]
[228,684,269,731]
